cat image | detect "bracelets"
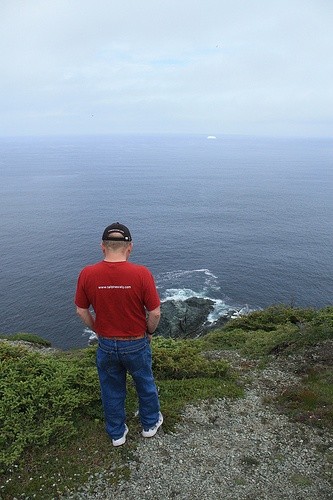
[147,331,155,335]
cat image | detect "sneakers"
[112,424,128,446]
[142,411,163,437]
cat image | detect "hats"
[102,222,132,241]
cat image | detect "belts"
[103,332,145,340]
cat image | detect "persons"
[75,222,164,447]
